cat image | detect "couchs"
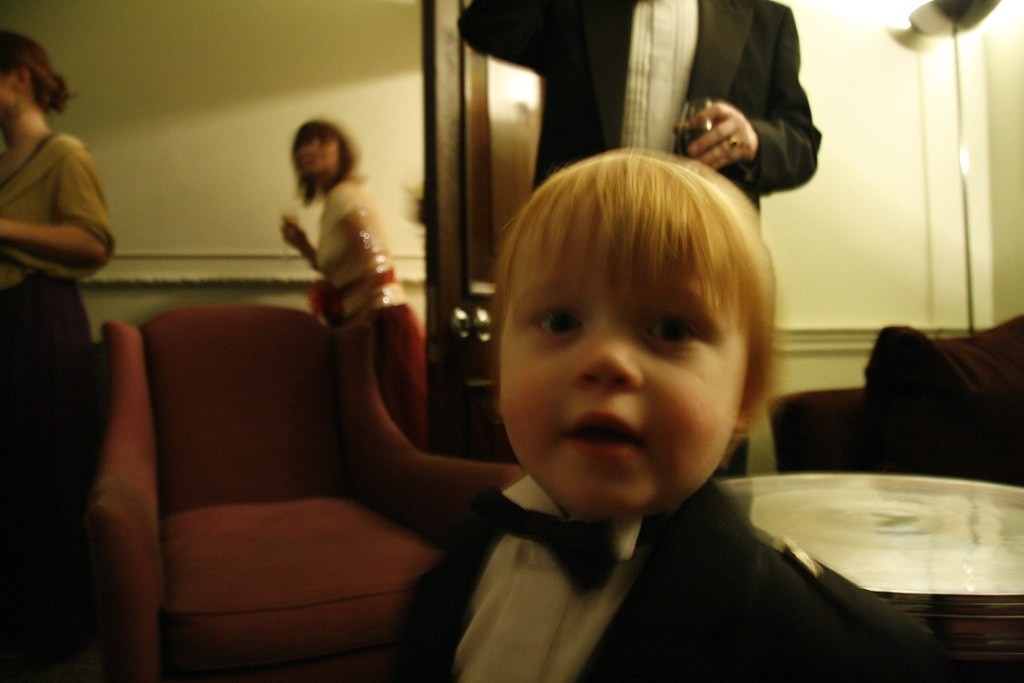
[84,299,527,683]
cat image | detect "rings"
[729,136,739,149]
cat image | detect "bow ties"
[458,482,618,592]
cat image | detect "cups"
[674,99,714,161]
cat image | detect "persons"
[377,146,955,683]
[0,30,121,683]
[453,0,827,479]
[278,116,433,498]
